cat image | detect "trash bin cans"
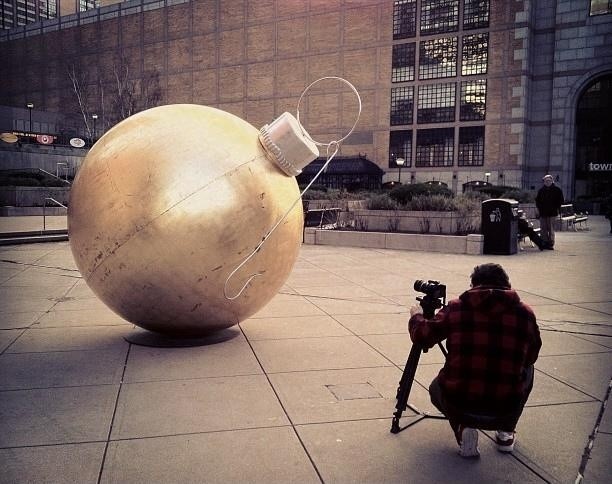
[482,198,518,255]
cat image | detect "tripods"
[390,301,448,433]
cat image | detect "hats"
[542,174,553,181]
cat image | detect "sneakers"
[456,423,480,457]
[494,430,517,451]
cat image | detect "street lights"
[92,113,99,144]
[27,102,35,144]
[485,170,491,182]
[395,157,406,186]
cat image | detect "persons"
[407,261,542,459]
[534,174,564,246]
[517,210,553,252]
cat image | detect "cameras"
[414,280,446,299]
